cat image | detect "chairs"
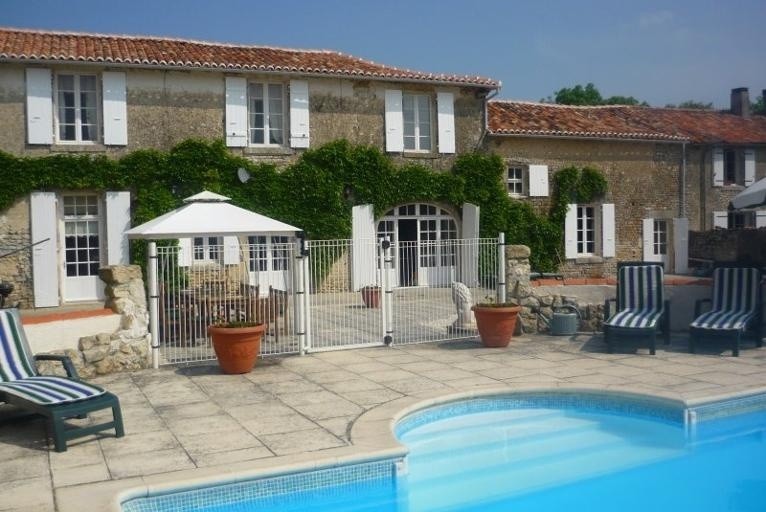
[0,308,124,452]
[688,261,766,357]
[603,261,671,355]
[159,281,288,347]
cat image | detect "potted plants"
[472,294,522,347]
[362,285,382,307]
[208,322,268,374]
